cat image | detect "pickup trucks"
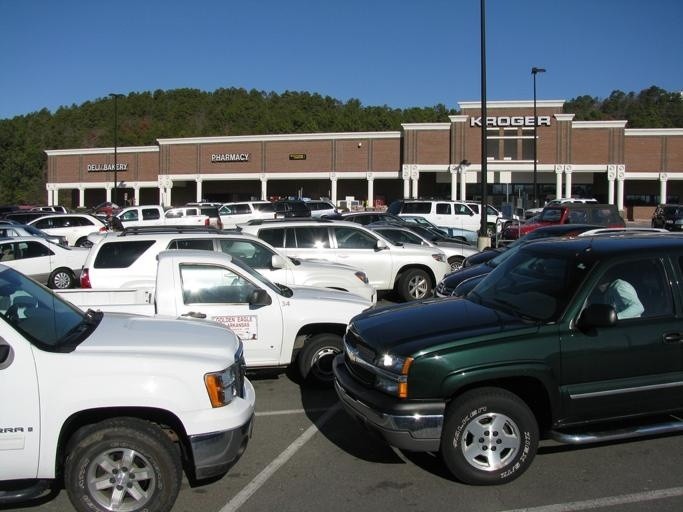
[43,247,370,386]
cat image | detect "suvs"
[328,227,683,487]
[79,226,378,306]
[0,261,260,510]
[226,217,451,302]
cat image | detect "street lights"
[529,64,545,199]
[108,91,122,204]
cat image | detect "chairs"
[624,261,670,318]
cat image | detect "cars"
[1,200,340,254]
[651,204,683,232]
[1,235,91,289]
[334,200,627,299]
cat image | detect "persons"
[581,268,644,322]
